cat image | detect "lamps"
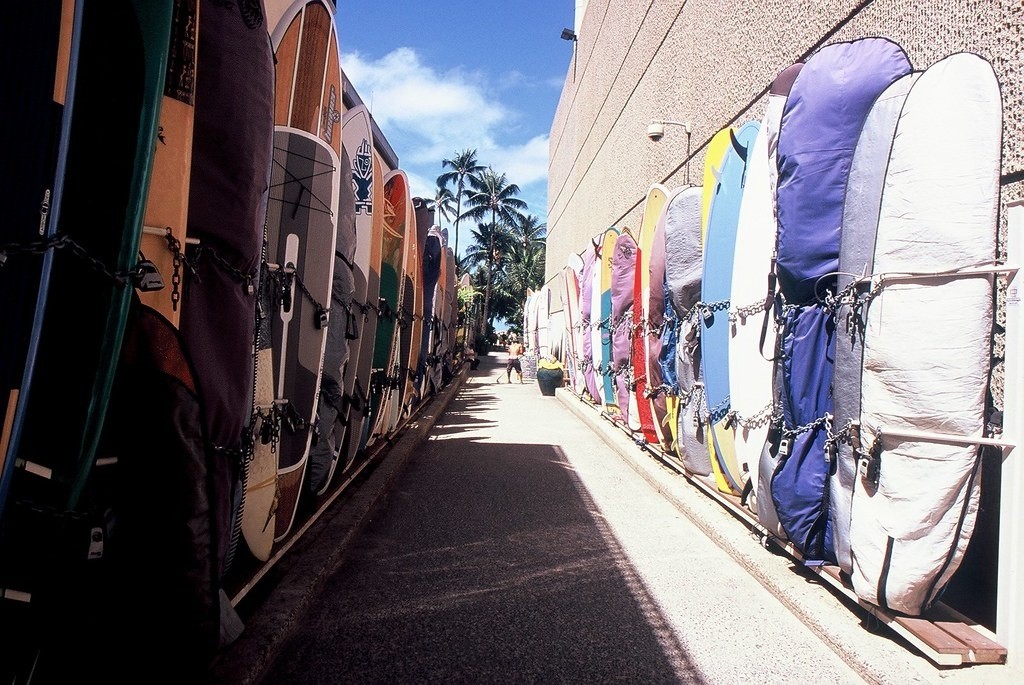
[560,28,578,41]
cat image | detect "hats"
[510,338,519,342]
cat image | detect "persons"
[499,332,506,347]
[464,343,480,370]
[507,337,524,385]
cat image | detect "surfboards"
[521,29,1004,617]
[0,0,473,608]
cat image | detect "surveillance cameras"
[647,124,665,142]
